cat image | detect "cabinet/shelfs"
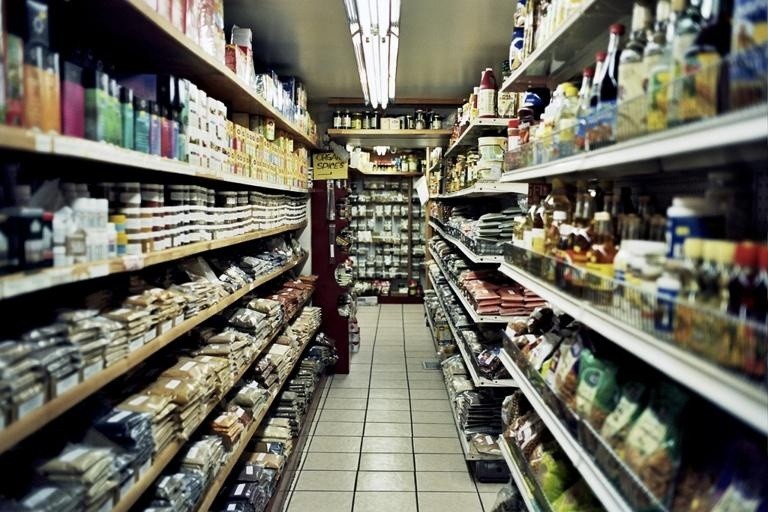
[315,178,361,376]
[0,2,321,512]
[352,178,425,304]
[430,2,768,509]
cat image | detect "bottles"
[506,118,522,170]
[576,0,730,151]
[13,185,31,207]
[398,153,418,173]
[539,172,611,305]
[471,66,497,119]
[611,169,768,382]
[53,182,127,267]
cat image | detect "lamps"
[342,0,401,113]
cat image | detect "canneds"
[352,112,362,129]
